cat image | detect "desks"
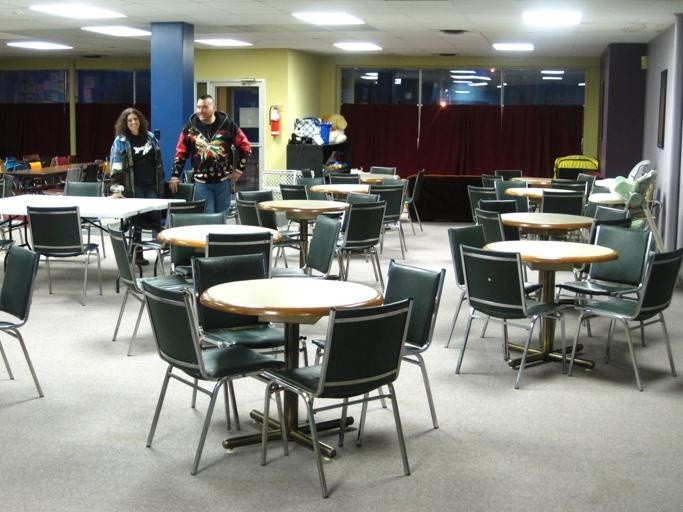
[483,237,618,376]
[156,223,283,247]
[0,193,187,294]
[199,276,386,461]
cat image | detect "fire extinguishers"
[269,105,281,137]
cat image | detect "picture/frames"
[656,68,667,148]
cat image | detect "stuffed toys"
[293,113,349,145]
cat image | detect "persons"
[168,94,252,226]
[107,105,167,267]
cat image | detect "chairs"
[108,228,211,357]
[568,247,682,392]
[65,179,107,260]
[234,162,429,293]
[203,228,274,278]
[140,277,291,479]
[467,153,662,281]
[190,252,311,431]
[453,244,567,390]
[24,206,103,306]
[0,177,33,252]
[2,242,45,410]
[445,223,546,375]
[0,154,114,198]
[132,198,207,277]
[260,294,418,498]
[552,225,653,349]
[306,257,446,449]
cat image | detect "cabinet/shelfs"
[286,140,351,184]
